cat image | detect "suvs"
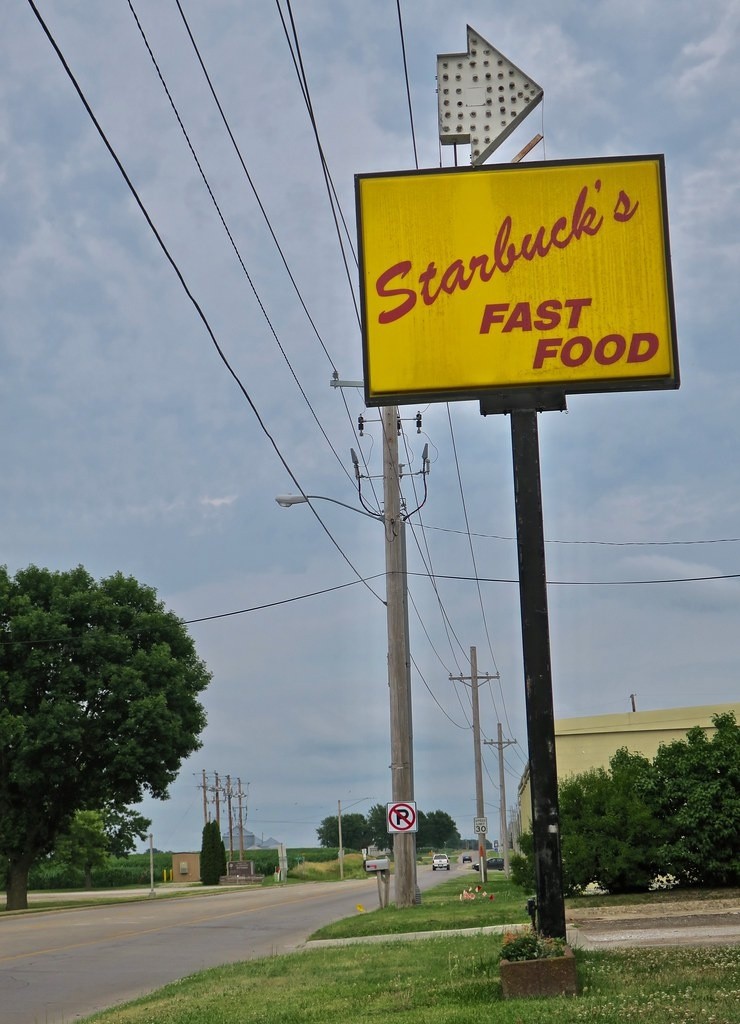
[432,854,451,871]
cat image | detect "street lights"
[473,798,510,879]
[277,494,419,906]
[337,797,376,879]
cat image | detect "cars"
[472,857,505,871]
[463,856,472,863]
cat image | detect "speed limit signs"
[474,817,488,834]
